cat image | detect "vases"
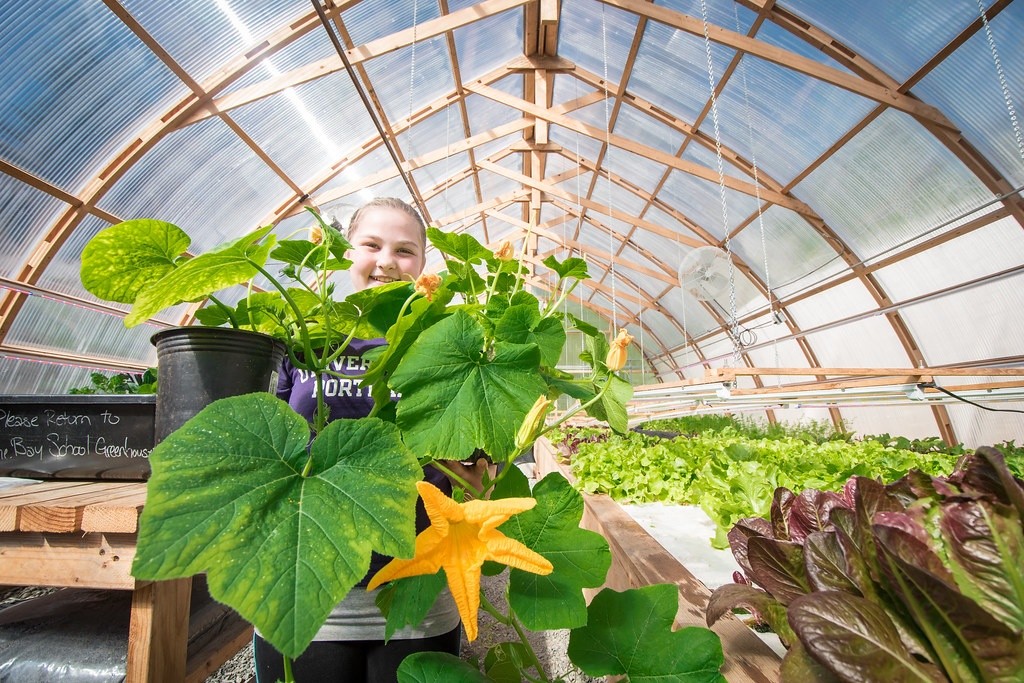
[0,393,156,481]
[150,326,286,448]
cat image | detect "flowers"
[81,205,729,683]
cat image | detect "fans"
[678,246,734,301]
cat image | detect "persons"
[253,198,498,683]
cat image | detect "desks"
[0,477,256,683]
